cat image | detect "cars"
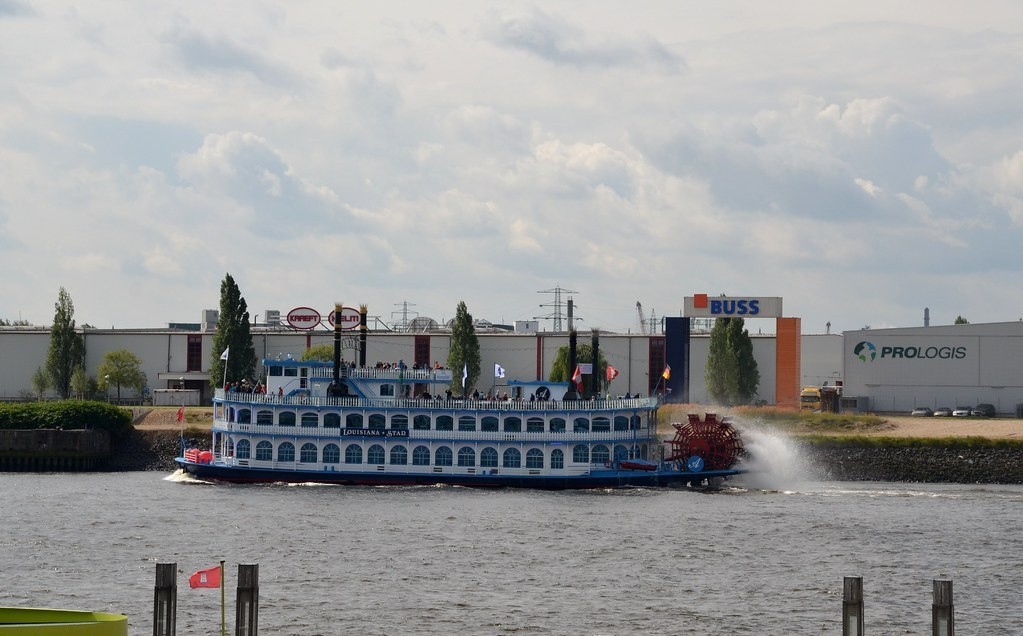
[912,407,933,417]
[934,407,952,417]
[952,406,971,417]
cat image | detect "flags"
[189,565,221,589]
[219,349,229,359]
[606,365,619,381]
[495,363,505,378]
[662,364,671,380]
[176,406,184,422]
[571,364,584,393]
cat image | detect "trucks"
[800,385,823,412]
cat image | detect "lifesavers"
[604,460,612,468]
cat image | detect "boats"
[174,350,754,486]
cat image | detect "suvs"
[971,404,996,418]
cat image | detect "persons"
[224,379,283,404]
[414,387,640,410]
[345,359,440,379]
